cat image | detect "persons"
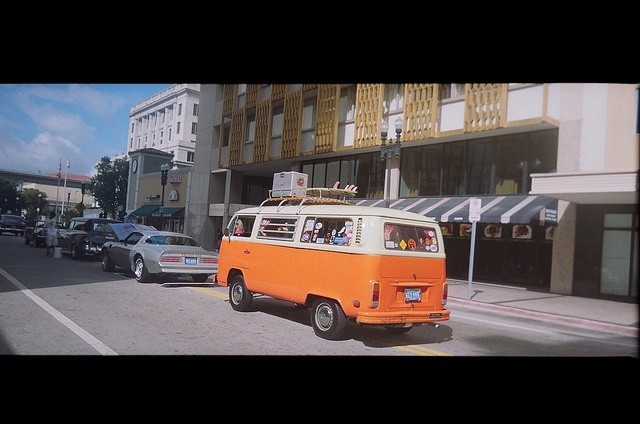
[43,211,58,258]
[234,219,245,236]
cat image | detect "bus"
[217,204,450,341]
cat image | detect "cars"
[0,214,26,236]
[56,216,123,259]
[100,223,219,283]
[25,221,65,246]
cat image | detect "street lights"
[159,163,169,231]
[379,117,403,209]
